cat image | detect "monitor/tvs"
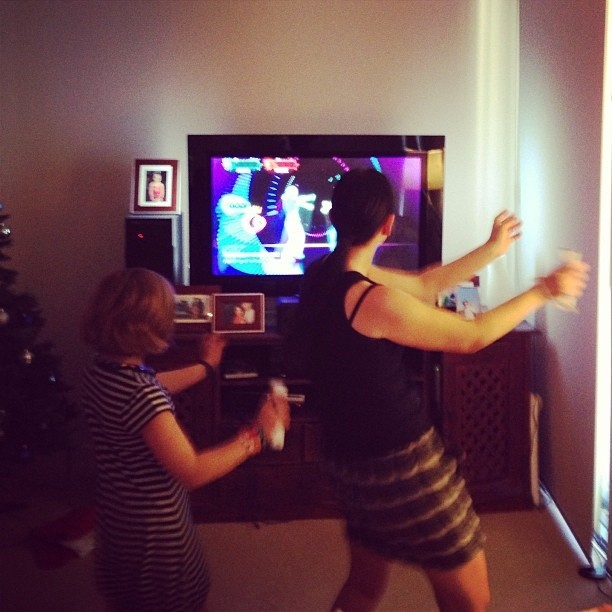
[188,135,446,304]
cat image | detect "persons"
[300,166,591,611]
[242,303,256,324]
[232,306,245,325]
[191,298,206,319]
[76,267,292,612]
[148,172,165,201]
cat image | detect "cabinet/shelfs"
[437,330,543,506]
[222,333,348,520]
[147,330,222,524]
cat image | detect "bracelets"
[200,360,213,375]
[254,424,269,455]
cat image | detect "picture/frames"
[211,292,265,335]
[171,293,212,324]
[455,287,482,318]
[128,156,182,216]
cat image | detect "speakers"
[124,213,182,285]
